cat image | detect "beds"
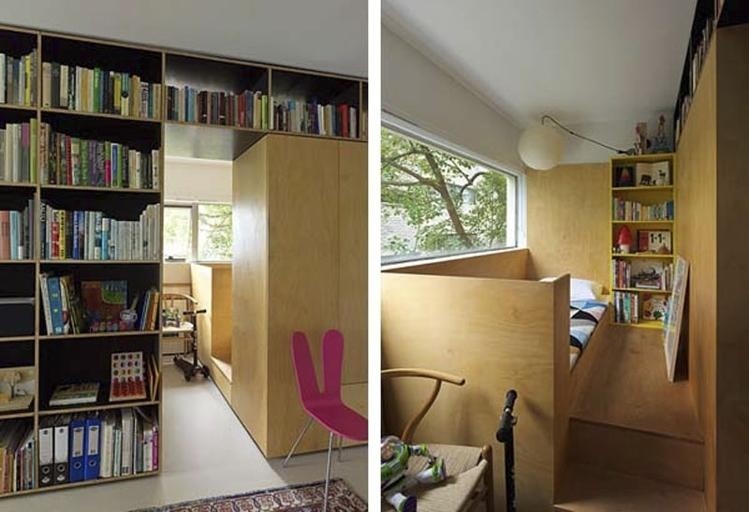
[381,247,612,509]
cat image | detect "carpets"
[138,478,367,509]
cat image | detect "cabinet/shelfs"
[231,132,368,463]
[0,28,162,494]
[162,49,369,142]
[611,156,676,329]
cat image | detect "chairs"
[381,367,493,510]
[281,328,367,512]
[159,290,197,380]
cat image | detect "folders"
[38,412,101,488]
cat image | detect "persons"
[163,304,184,327]
[380,431,447,512]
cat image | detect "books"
[613,197,676,323]
[0,45,164,497]
[165,83,360,138]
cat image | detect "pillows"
[538,276,603,300]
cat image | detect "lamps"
[518,115,635,173]
